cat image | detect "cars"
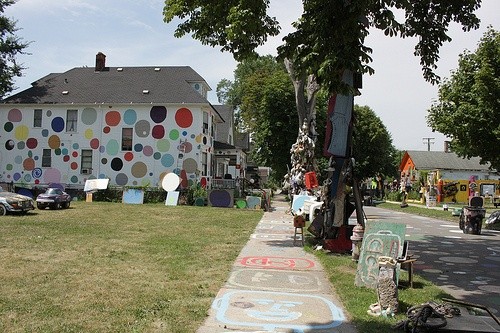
[0,186,36,216]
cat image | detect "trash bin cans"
[460,206,486,234]
[364,193,372,206]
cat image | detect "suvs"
[37,188,71,209]
